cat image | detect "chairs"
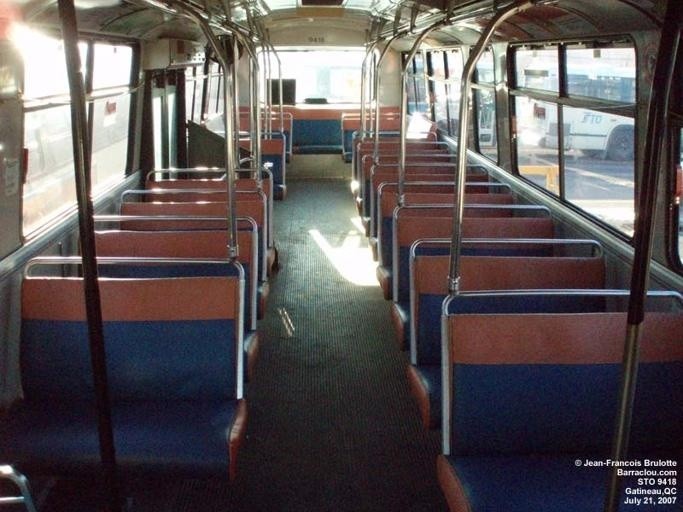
[0,110,292,512]
[340,112,682,512]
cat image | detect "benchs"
[238,106,399,154]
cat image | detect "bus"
[518,63,636,162]
